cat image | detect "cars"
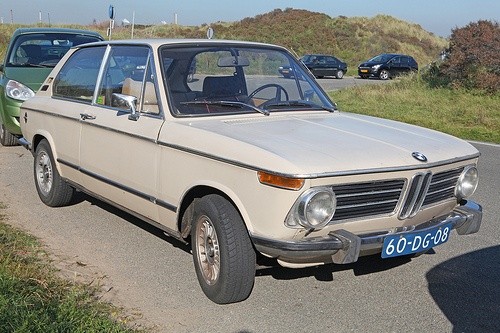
[0,27,128,147]
[277,54,347,81]
[122,55,197,83]
[19,39,484,305]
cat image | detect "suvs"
[358,53,418,80]
